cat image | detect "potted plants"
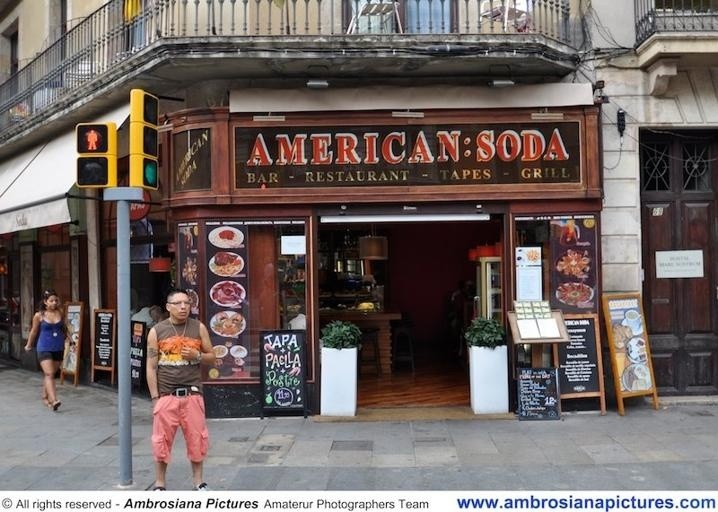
[314,319,363,417]
[462,317,509,415]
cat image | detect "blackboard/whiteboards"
[130,320,147,388]
[260,329,307,415]
[553,313,604,399]
[91,308,116,371]
[518,367,563,421]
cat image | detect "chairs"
[347,0,404,34]
[479,1,533,34]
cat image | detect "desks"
[318,311,402,379]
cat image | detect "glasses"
[44,290,55,295]
[167,301,190,305]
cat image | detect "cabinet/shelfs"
[475,257,504,328]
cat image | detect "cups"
[626,310,641,328]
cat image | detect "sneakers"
[193,482,208,491]
[152,487,165,491]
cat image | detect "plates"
[207,225,248,358]
[623,338,651,392]
[556,283,594,306]
[622,321,644,336]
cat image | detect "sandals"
[41,397,52,408]
[52,399,61,411]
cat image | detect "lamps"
[306,65,330,90]
[489,64,516,87]
[357,222,390,261]
[148,256,171,273]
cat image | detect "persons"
[24,289,77,411]
[146,289,216,491]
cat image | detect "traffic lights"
[74,122,118,188]
[129,88,159,192]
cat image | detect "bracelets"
[70,342,75,346]
[197,351,202,361]
[151,396,160,401]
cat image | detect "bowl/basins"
[553,250,592,282]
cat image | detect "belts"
[171,388,203,396]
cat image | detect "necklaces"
[169,318,187,344]
